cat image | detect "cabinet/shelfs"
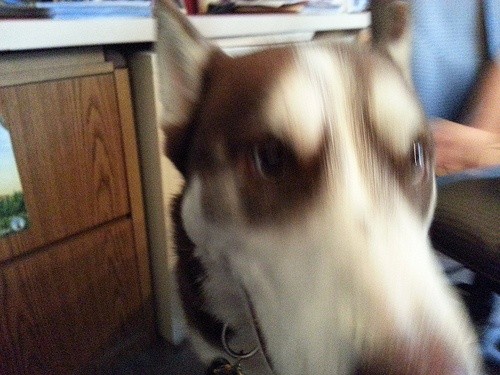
[0,45,156,375]
[128,25,354,349]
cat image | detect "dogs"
[151,0,488,374]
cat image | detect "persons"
[365,0,500,290]
